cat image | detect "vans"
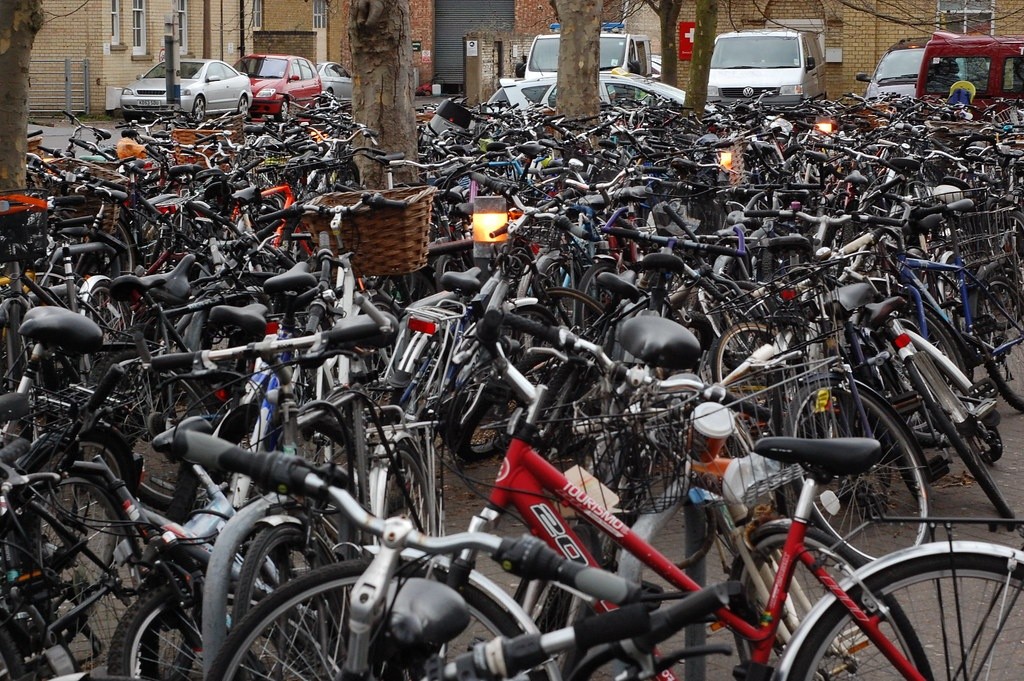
[914,30,1024,123]
[515,22,652,82]
[705,28,826,107]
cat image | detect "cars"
[314,62,353,99]
[231,54,322,124]
[856,36,967,100]
[482,67,718,115]
[120,58,253,124]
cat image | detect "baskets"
[173,112,243,173]
[855,103,890,133]
[952,207,1013,266]
[704,259,836,365]
[997,106,1018,127]
[535,400,694,517]
[509,200,566,248]
[31,154,129,239]
[304,181,438,274]
[907,180,990,248]
[644,356,872,526]
[718,139,746,184]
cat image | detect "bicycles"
[0,86,1024,681]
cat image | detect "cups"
[690,401,735,462]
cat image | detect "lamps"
[721,152,733,169]
[816,116,831,134]
[474,194,507,259]
